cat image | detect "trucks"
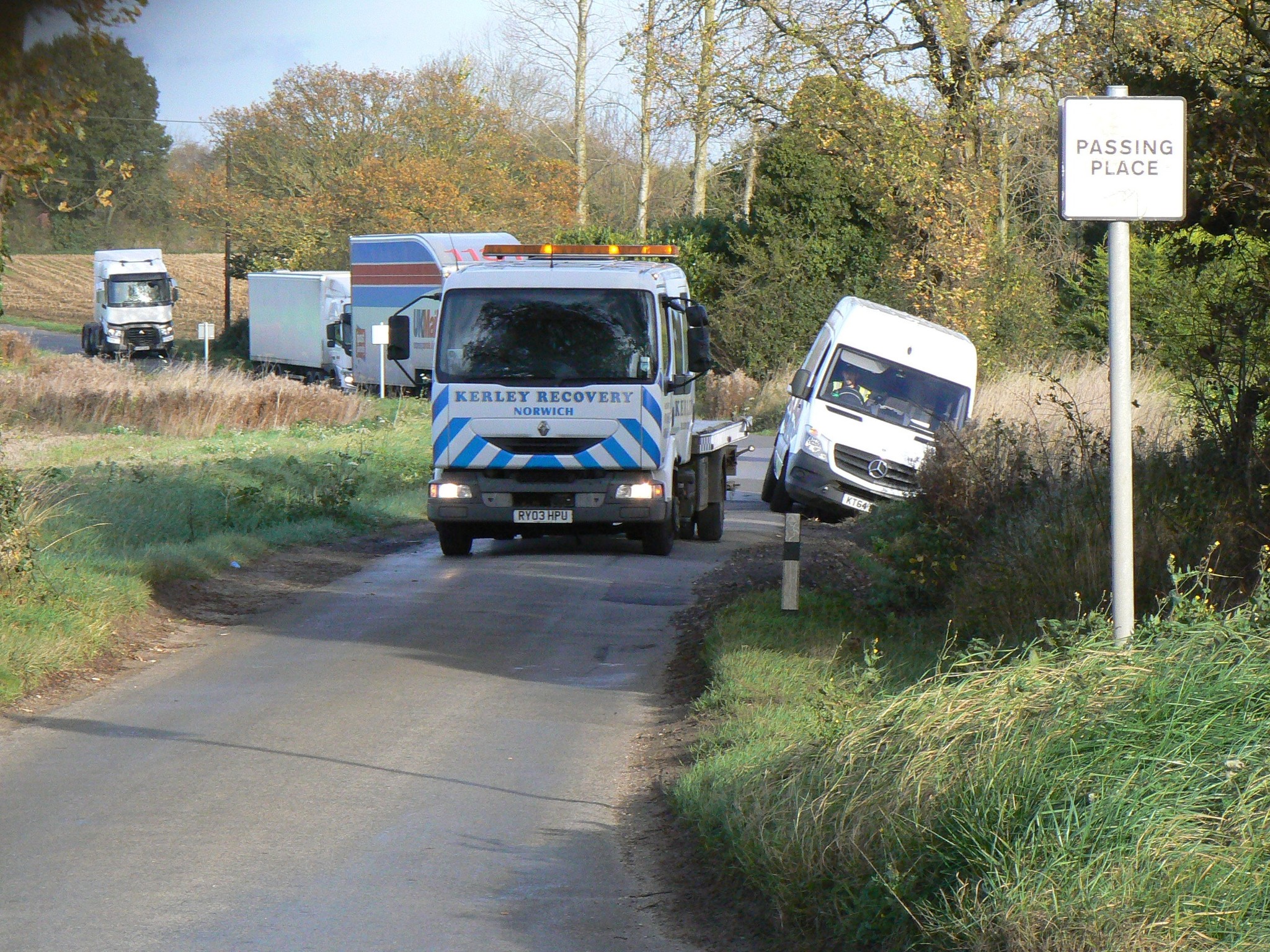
[247,233,752,555]
[82,249,178,361]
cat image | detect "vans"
[761,297,977,524]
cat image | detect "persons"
[825,360,873,408]
